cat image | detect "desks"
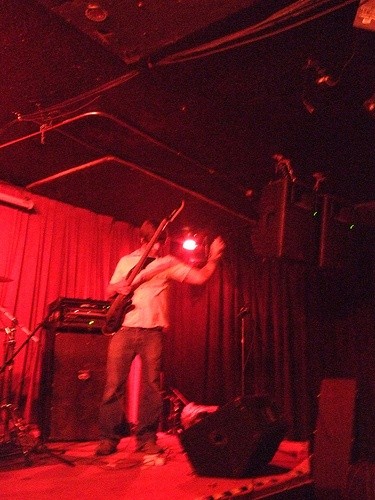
[40,323,130,441]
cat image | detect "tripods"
[0,307,72,467]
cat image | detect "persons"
[95,219,225,456]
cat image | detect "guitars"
[102,198,186,337]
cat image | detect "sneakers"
[138,432,164,454]
[96,437,117,455]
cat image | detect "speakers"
[180,395,290,478]
[40,330,130,440]
[255,178,318,264]
[312,193,358,276]
